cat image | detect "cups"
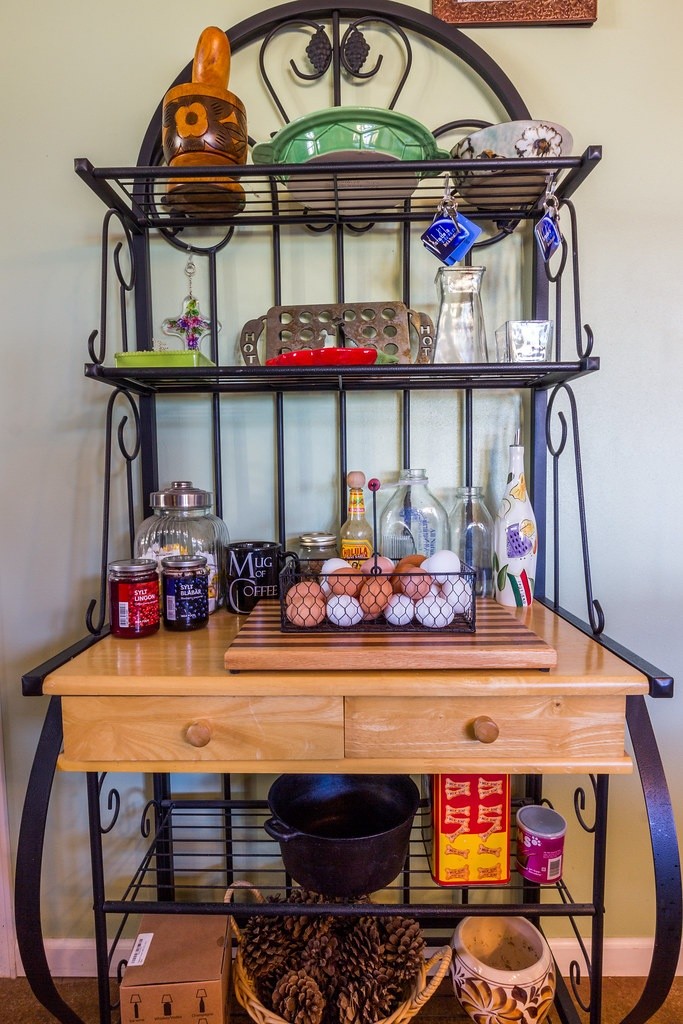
[493,318,554,362]
[223,540,300,616]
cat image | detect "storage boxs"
[119,913,230,1023]
[420,774,511,886]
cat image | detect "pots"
[263,774,421,899]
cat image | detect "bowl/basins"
[449,119,573,211]
[253,106,451,217]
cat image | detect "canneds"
[162,554,210,633]
[297,532,340,584]
[515,804,567,885]
[106,558,161,640]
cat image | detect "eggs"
[287,550,472,629]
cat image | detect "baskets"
[224,880,451,1024]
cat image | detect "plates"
[265,347,378,367]
[113,350,218,368]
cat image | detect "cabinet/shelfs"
[14,0,683,1024]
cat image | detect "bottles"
[296,530,338,582]
[430,266,490,364]
[448,915,557,1024]
[493,445,539,607]
[379,468,448,565]
[448,486,493,597]
[338,471,373,569]
[134,481,230,615]
